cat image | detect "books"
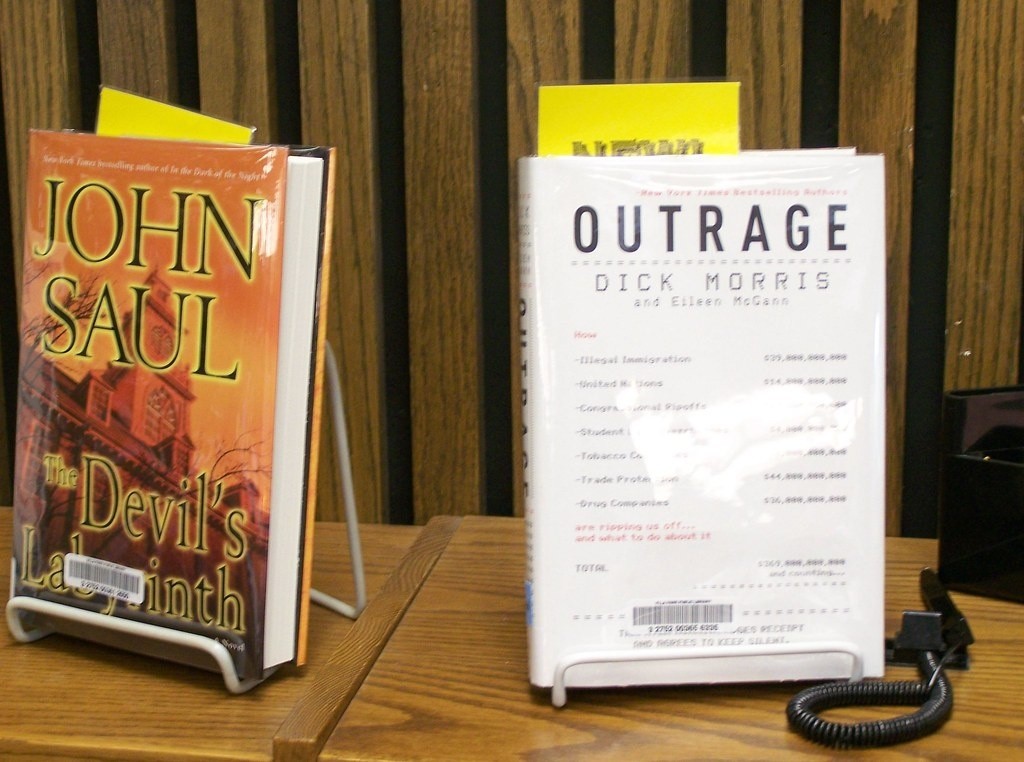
[6,124,339,686]
[516,146,888,691]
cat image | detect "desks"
[273,514,1024,762]
[0,506,423,762]
